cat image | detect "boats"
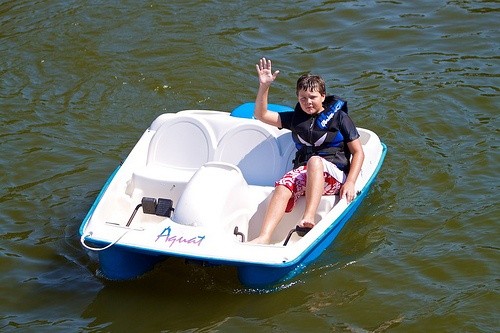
[79,101,388,290]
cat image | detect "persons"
[244,57,365,245]
